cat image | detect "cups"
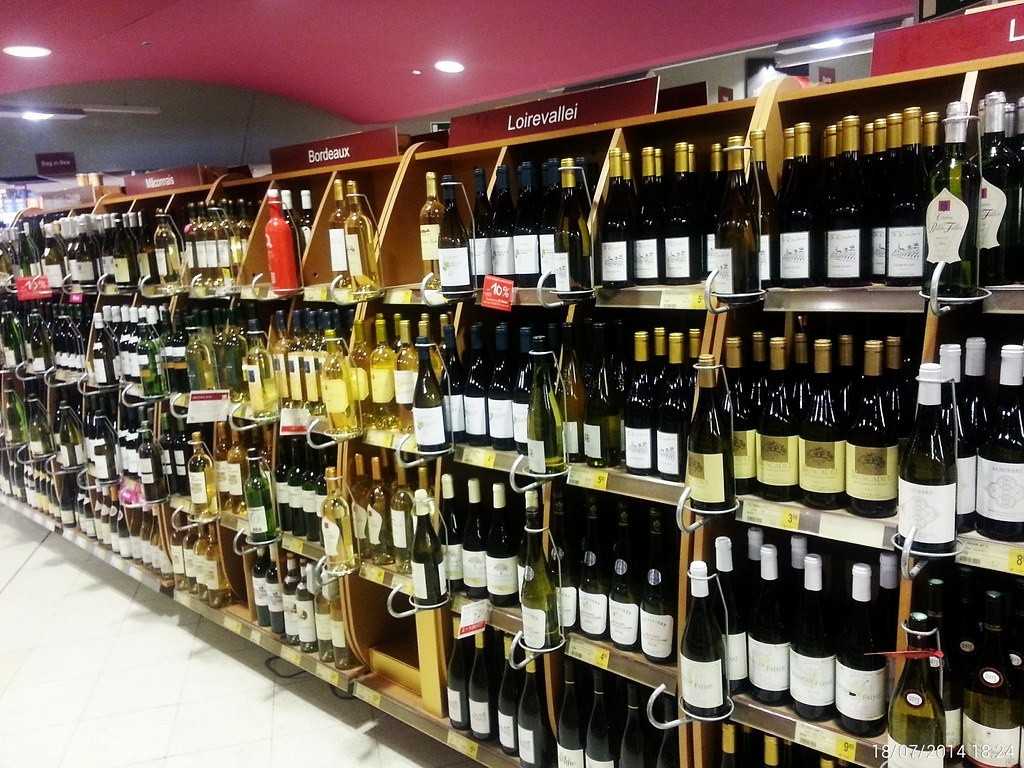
[75,172,104,187]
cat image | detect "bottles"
[139,407,339,545]
[0,183,81,214]
[557,656,679,768]
[159,302,357,425]
[421,141,703,299]
[678,105,946,737]
[154,179,387,300]
[447,614,558,768]
[719,720,871,768]
[922,90,1024,304]
[888,578,1024,768]
[319,311,916,520]
[0,211,158,574]
[156,504,231,610]
[897,336,1024,560]
[130,169,136,176]
[252,546,359,669]
[321,447,681,667]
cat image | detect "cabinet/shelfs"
[0,49,1024,768]
